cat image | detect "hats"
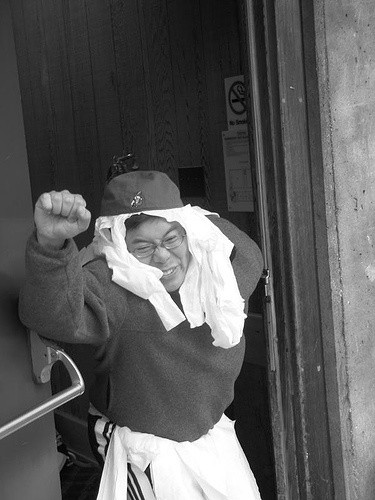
[98,170,183,218]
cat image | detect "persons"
[18,170,264,500]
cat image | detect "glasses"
[128,229,186,259]
